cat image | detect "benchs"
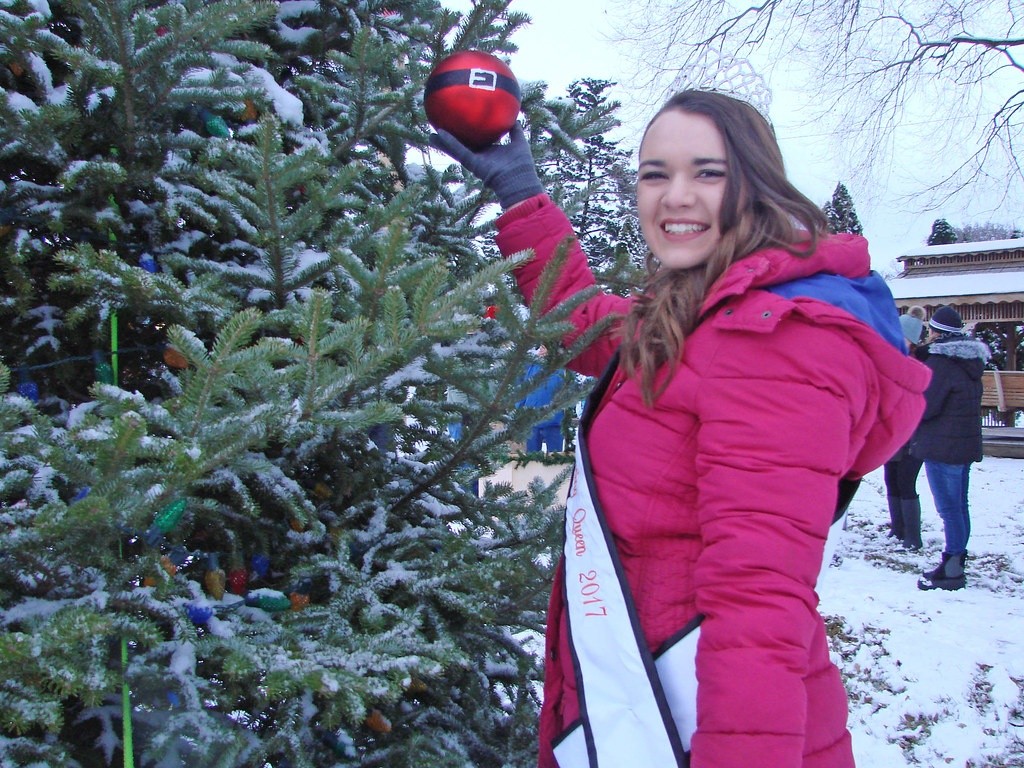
[981,371,1024,438]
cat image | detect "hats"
[929,304,963,333]
[899,305,928,346]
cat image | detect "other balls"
[423,48,522,148]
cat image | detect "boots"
[917,551,967,590]
[884,495,924,552]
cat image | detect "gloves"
[430,120,542,209]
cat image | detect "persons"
[430,90,933,768]
[516,344,566,453]
[884,306,989,591]
